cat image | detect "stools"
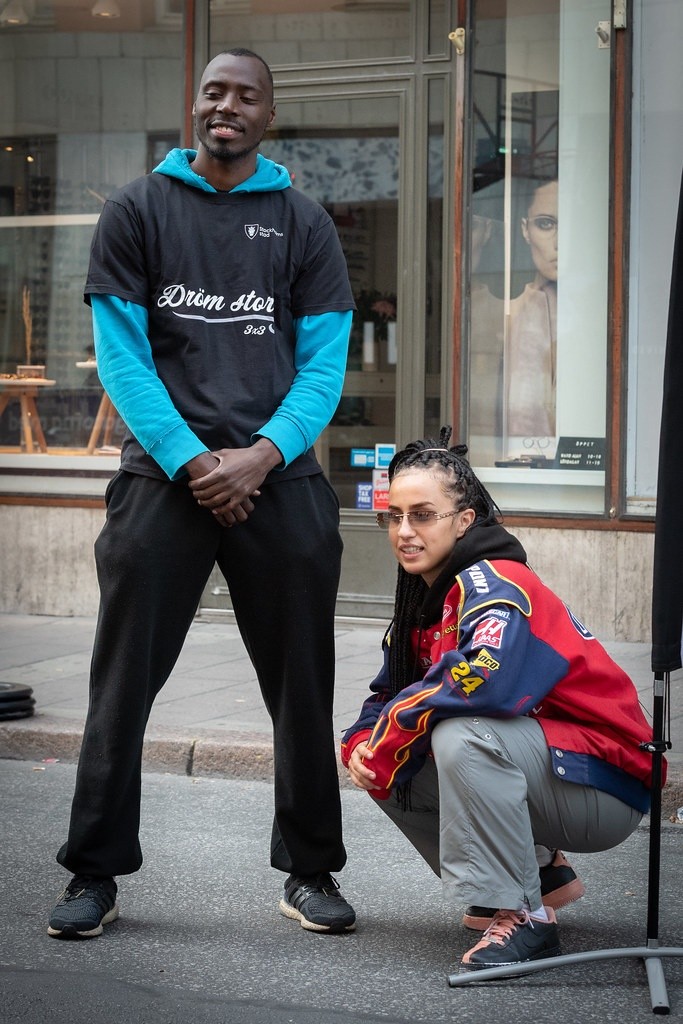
[0,376,57,453]
[76,362,116,454]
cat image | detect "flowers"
[349,288,396,340]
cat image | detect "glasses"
[376,508,466,530]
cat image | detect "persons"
[466,153,558,435]
[46,49,360,940]
[340,425,670,968]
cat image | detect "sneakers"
[463,850,586,930]
[462,905,561,966]
[48,876,120,936]
[278,873,359,933]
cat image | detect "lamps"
[91,0,121,19]
[0,0,29,26]
